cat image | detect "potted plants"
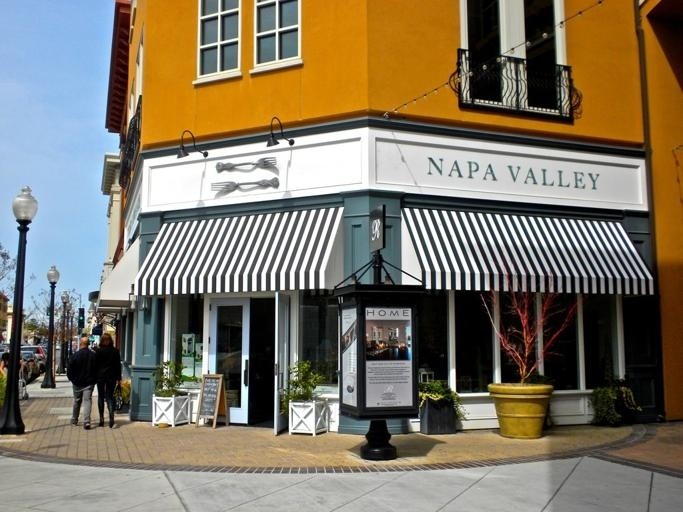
[114,377,130,413]
[416,378,470,434]
[588,376,643,427]
[478,271,578,439]
[278,360,330,437]
[150,360,193,427]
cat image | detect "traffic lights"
[78,307,84,329]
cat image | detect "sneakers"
[72,419,77,426]
[84,422,90,430]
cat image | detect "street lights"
[55,290,75,374]
[0,185,37,435]
[39,264,59,388]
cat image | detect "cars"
[0,342,77,387]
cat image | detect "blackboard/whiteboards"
[197,374,222,418]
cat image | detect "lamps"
[265,116,295,148]
[128,284,152,312]
[176,129,208,159]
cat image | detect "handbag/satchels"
[113,385,123,410]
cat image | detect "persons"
[95,333,123,428]
[67,336,98,430]
[0,353,9,412]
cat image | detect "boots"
[99,417,104,426]
[110,415,114,428]
